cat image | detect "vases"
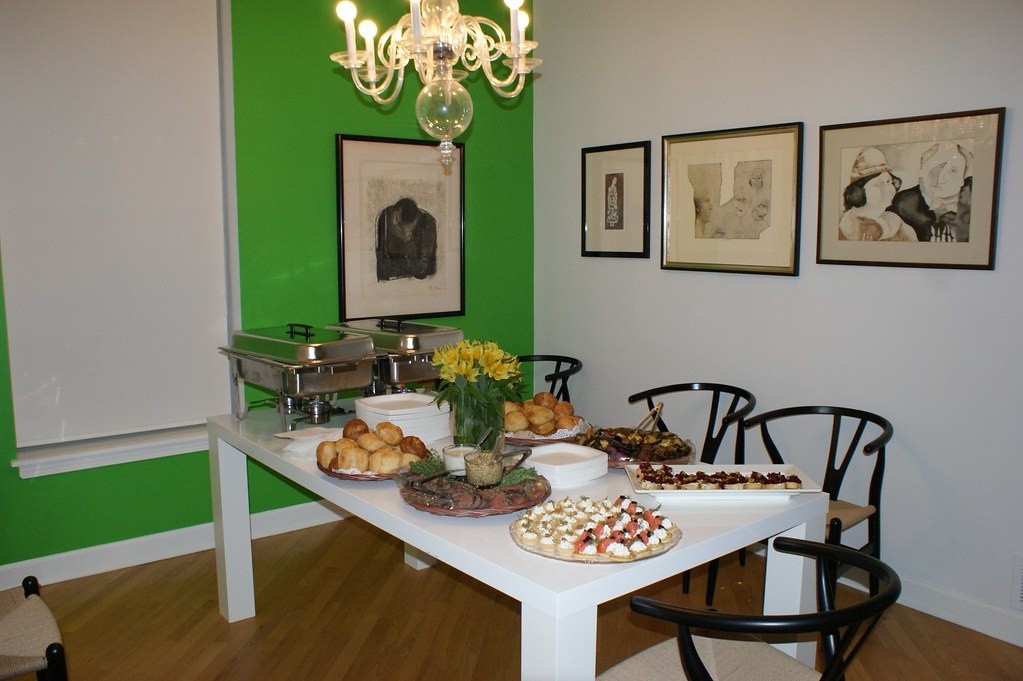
[448,384,504,454]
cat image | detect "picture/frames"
[581,140,651,257]
[333,132,465,323]
[659,121,804,276]
[815,107,1007,270]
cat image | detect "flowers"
[427,338,524,451]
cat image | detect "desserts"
[637,461,802,490]
[514,494,677,561]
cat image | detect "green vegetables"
[408,447,540,487]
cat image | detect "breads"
[316,419,428,474]
[503,392,585,436]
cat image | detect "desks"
[209,397,831,681]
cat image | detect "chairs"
[625,383,758,590]
[510,354,582,405]
[702,405,892,605]
[594,537,901,681]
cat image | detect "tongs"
[628,401,663,435]
[406,468,466,499]
[496,449,533,479]
[598,430,639,455]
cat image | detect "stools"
[0,575,67,681]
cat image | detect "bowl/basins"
[442,443,480,476]
[464,450,504,489]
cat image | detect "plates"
[623,464,823,501]
[512,442,608,487]
[355,392,451,446]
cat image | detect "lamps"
[330,0,545,168]
